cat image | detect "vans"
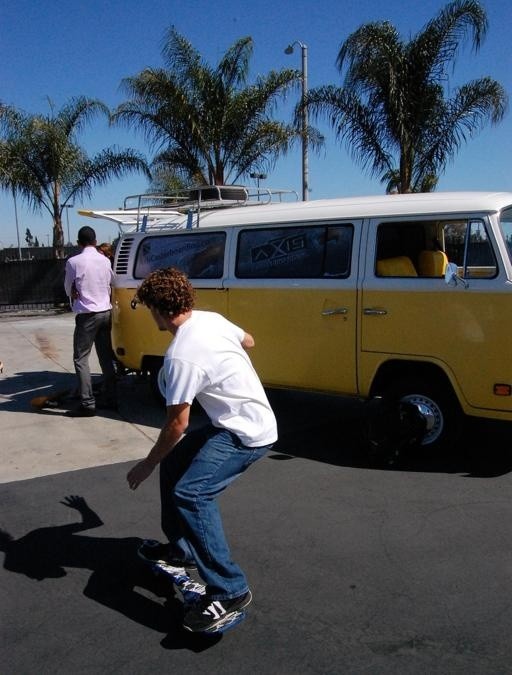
[78,186,511,464]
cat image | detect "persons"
[97,242,127,379]
[61,223,124,417]
[126,266,282,634]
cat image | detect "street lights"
[283,38,310,202]
[250,173,267,201]
[61,203,75,247]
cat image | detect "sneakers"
[183,589,252,632]
[138,543,198,571]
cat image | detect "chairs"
[376,246,449,278]
